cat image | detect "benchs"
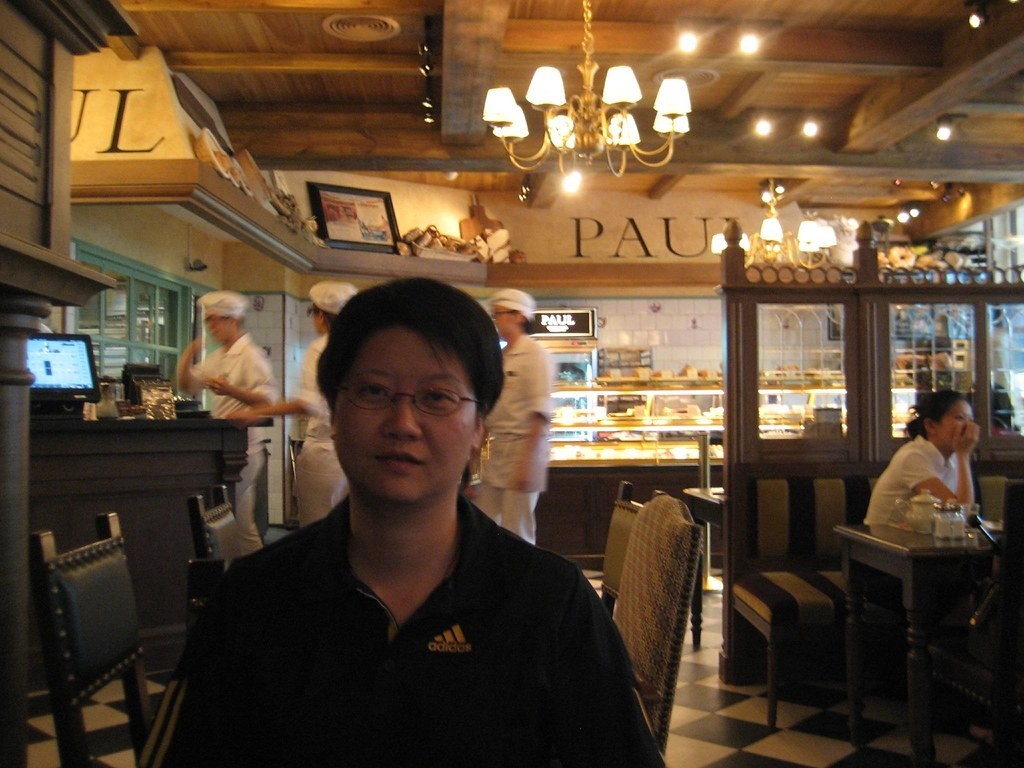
[725,458,1010,732]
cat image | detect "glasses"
[491,308,516,319]
[339,379,476,416]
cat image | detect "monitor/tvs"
[25,331,101,415]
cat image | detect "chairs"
[31,511,155,768]
[191,484,247,570]
[598,481,703,756]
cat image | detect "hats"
[195,291,249,319]
[307,280,359,315]
[487,288,537,323]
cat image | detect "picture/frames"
[306,179,403,255]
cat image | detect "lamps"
[518,176,533,203]
[481,0,690,176]
[415,15,442,123]
[712,180,838,271]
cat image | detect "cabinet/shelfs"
[531,379,919,571]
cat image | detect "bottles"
[932,503,964,540]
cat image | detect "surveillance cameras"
[193,258,208,272]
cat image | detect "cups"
[146,388,175,419]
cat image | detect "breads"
[603,364,722,379]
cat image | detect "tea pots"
[896,487,956,534]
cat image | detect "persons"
[138,279,665,768]
[466,287,552,546]
[176,293,277,558]
[864,389,980,702]
[229,280,357,529]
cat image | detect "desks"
[831,519,1002,768]
[681,485,725,651]
